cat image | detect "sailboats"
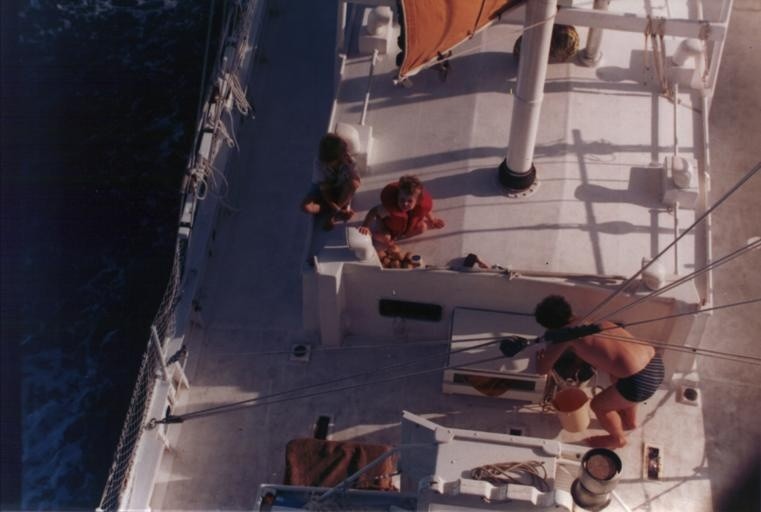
[94,1,734,512]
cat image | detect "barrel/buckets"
[552,388,590,432]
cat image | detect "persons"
[297,131,362,231]
[534,293,666,448]
[358,174,446,246]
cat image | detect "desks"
[441,306,549,405]
